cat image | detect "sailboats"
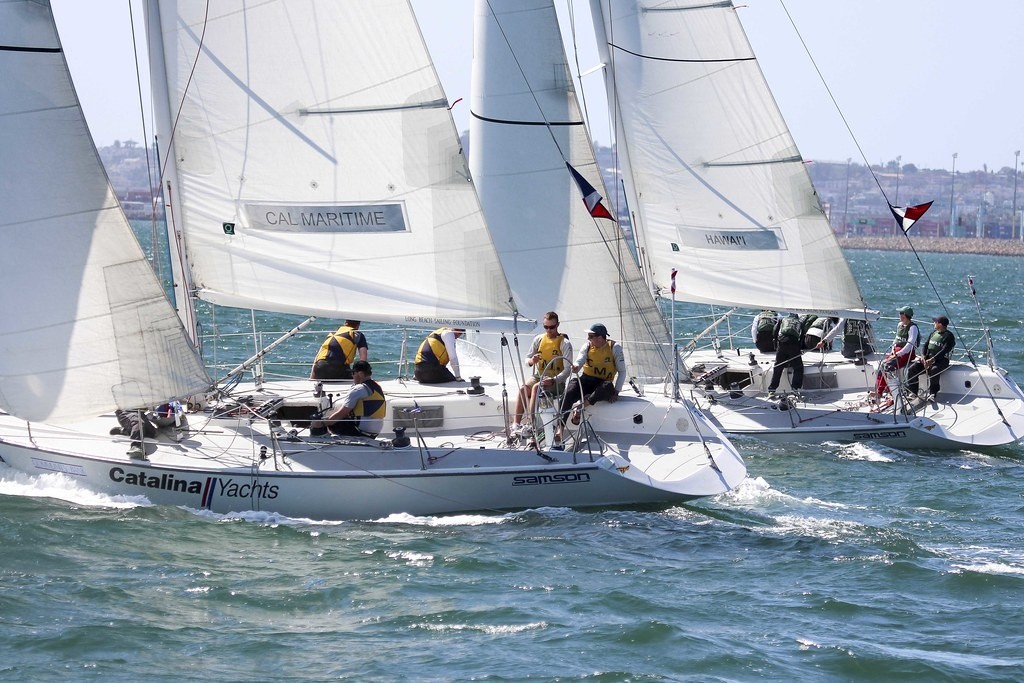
[467,0,1024,452]
[0,0,749,522]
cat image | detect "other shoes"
[514,422,533,436]
[927,395,935,403]
[571,410,581,424]
[509,423,523,439]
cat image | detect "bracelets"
[551,377,556,385]
[320,417,329,426]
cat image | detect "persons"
[554,323,626,441]
[509,311,573,439]
[310,360,386,439]
[752,309,784,352]
[413,327,466,384]
[908,315,956,402]
[768,313,806,400]
[817,317,873,358]
[868,305,921,404]
[110,401,186,458]
[310,320,368,379]
[803,316,839,351]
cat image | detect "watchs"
[821,340,826,344]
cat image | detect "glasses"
[353,372,355,375]
[900,312,904,315]
[589,335,595,339]
[544,322,558,329]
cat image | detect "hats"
[896,306,913,317]
[932,315,949,326]
[345,360,372,373]
[584,323,607,336]
